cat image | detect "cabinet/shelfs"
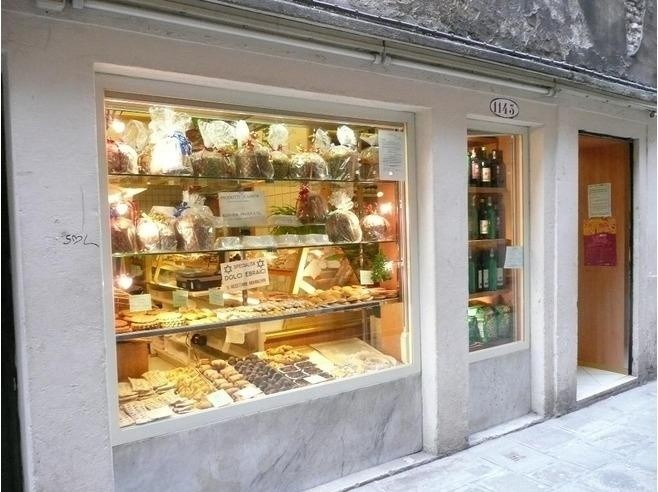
[466,118,530,364]
[102,93,423,448]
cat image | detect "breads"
[110,210,215,254]
[175,343,334,409]
[326,211,386,243]
[358,351,398,370]
[114,286,399,333]
[106,139,379,191]
[296,194,326,223]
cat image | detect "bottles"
[468,194,500,240]
[467,145,503,186]
[470,248,507,294]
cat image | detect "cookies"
[118,369,196,428]
